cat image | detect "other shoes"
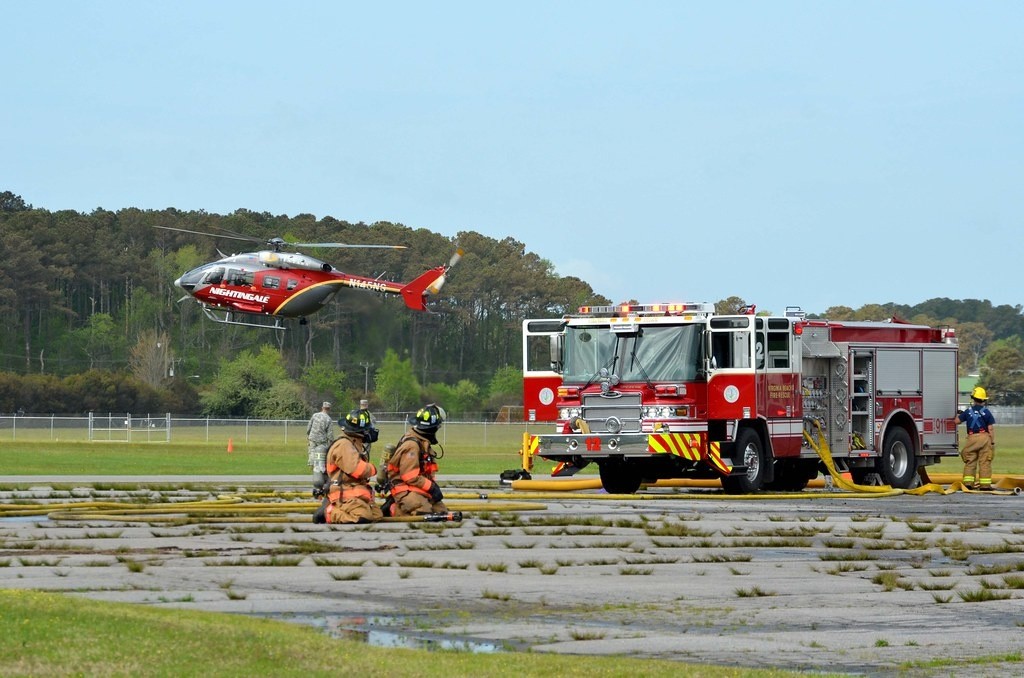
[312,496,331,524]
[380,494,395,517]
[966,485,973,490]
[980,487,997,491]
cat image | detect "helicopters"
[152,222,464,330]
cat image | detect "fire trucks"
[522,301,961,493]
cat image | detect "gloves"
[428,480,443,504]
[957,410,963,415]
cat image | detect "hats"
[323,402,331,408]
[360,400,369,405]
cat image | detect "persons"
[379,403,446,515]
[312,409,384,524]
[346,400,377,462]
[306,401,334,475]
[954,386,995,489]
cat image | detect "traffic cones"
[227,438,234,453]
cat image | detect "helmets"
[408,403,447,429]
[970,386,989,400]
[338,409,375,432]
